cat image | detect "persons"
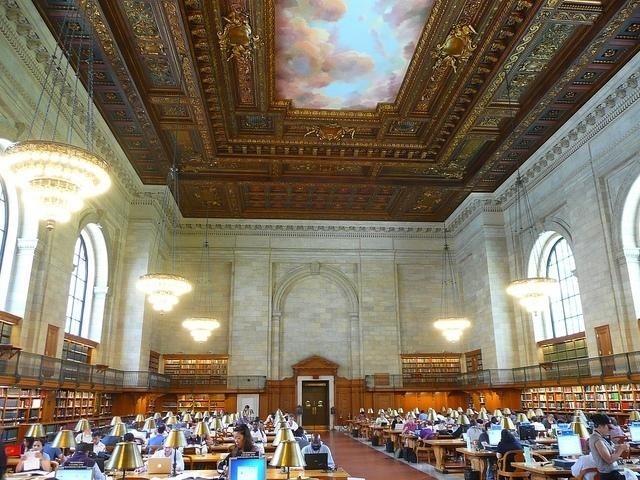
[16,406,335,476]
[356,408,639,479]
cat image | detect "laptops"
[54,469,96,480]
[148,457,171,474]
[304,453,330,469]
[380,422,387,427]
[229,456,266,480]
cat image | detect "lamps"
[432,210,471,343]
[183,172,219,343]
[504,70,559,315]
[0,0,114,229]
[138,58,195,315]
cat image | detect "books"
[1,388,94,426]
[520,386,640,417]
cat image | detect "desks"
[3,409,351,480]
[342,408,640,480]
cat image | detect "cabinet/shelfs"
[402,353,462,384]
[63,335,97,383]
[149,350,161,384]
[176,394,225,413]
[0,387,113,426]
[520,384,640,419]
[0,311,22,351]
[537,332,590,377]
[162,355,229,384]
[465,350,484,385]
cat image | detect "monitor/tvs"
[452,423,640,468]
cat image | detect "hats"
[592,413,617,429]
